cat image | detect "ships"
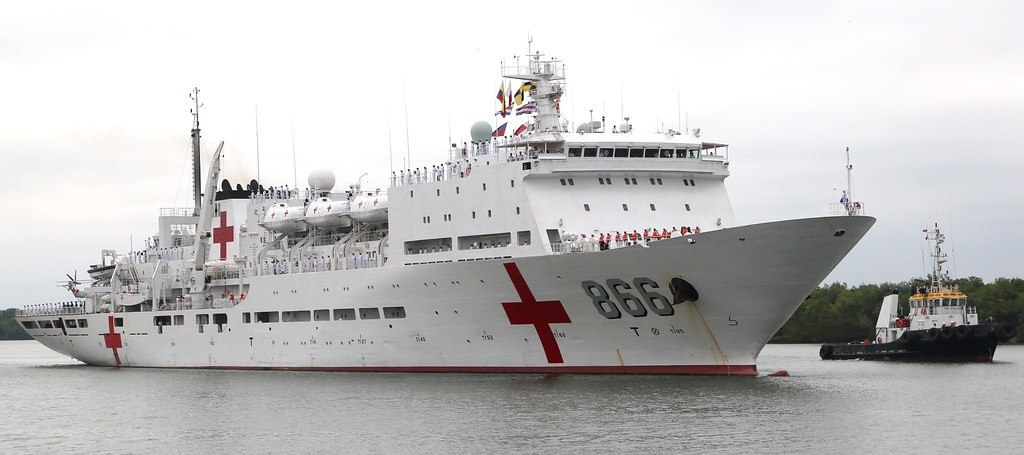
[14,36,877,378]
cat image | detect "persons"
[695,226,700,234]
[128,245,184,264]
[251,184,289,203]
[578,233,611,251]
[469,240,528,249]
[616,230,638,245]
[463,135,520,157]
[508,146,539,162]
[419,246,452,254]
[176,296,181,310]
[24,301,85,314]
[643,227,677,240]
[264,251,377,275]
[221,290,245,305]
[392,158,478,186]
[681,226,692,235]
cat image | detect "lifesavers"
[878,337,882,343]
[921,308,926,315]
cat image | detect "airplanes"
[57,269,91,298]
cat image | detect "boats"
[348,192,388,222]
[305,197,351,228]
[261,202,306,231]
[818,220,1014,363]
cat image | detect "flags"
[491,122,528,136]
[495,80,537,116]
